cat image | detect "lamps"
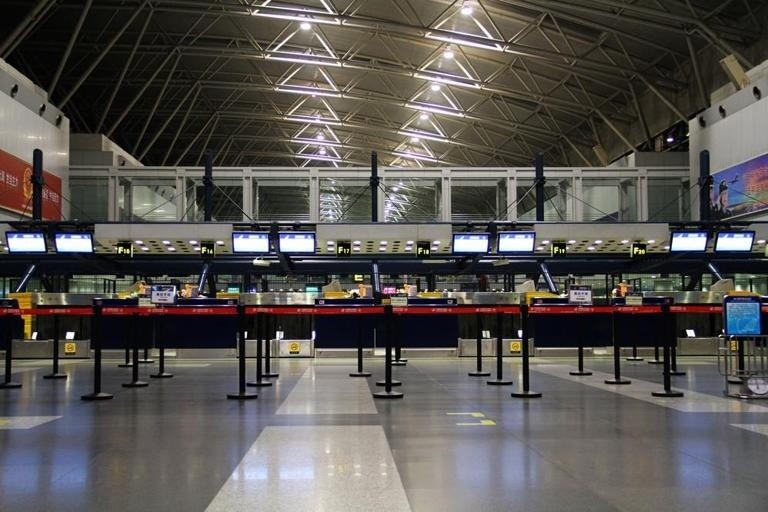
[420,0,471,119]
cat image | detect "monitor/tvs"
[451,231,491,256]
[231,230,270,255]
[277,231,315,256]
[496,230,536,255]
[669,228,707,252]
[53,230,94,255]
[5,230,48,253]
[714,229,757,254]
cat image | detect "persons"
[709,175,719,218]
[716,179,732,219]
